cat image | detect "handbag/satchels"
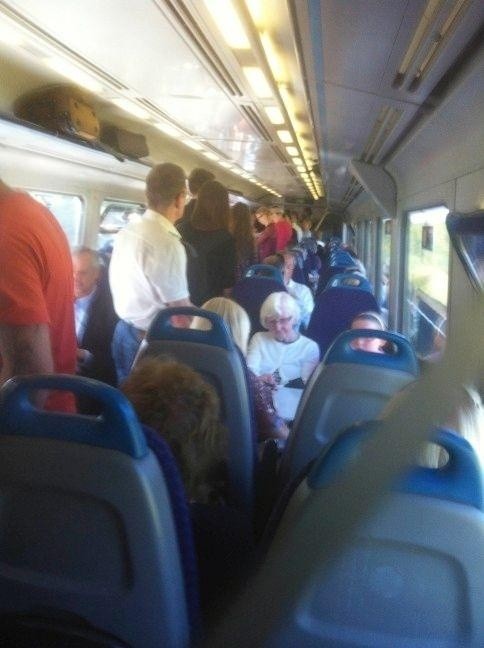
[15,89,100,140]
[104,127,149,159]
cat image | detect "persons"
[1,175,79,414]
[101,163,315,389]
[69,250,117,372]
[110,355,228,586]
[342,272,384,353]
[191,291,321,438]
